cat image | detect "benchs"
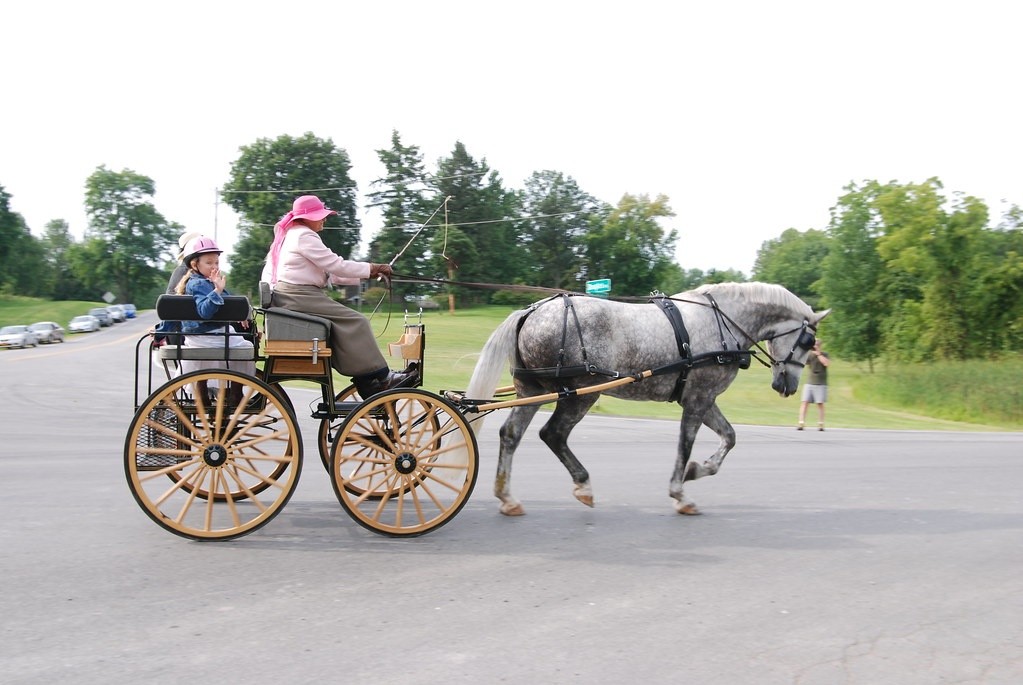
[155,294,258,405]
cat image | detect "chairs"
[259,281,331,348]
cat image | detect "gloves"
[370,263,393,278]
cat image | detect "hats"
[178,231,205,259]
[182,237,223,268]
[281,196,338,229]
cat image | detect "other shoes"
[818,422,824,431]
[350,371,419,401]
[246,392,262,409]
[797,421,803,429]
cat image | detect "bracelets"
[816,353,823,358]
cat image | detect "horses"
[464,282,831,517]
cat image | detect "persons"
[263,195,419,400]
[150,232,263,406]
[798,340,831,431]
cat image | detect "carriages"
[123,282,832,542]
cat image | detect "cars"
[107,305,126,323]
[68,315,100,334]
[123,304,136,318]
[347,297,367,305]
[90,308,113,326]
[0,324,38,348]
[29,322,64,343]
[404,295,415,302]
